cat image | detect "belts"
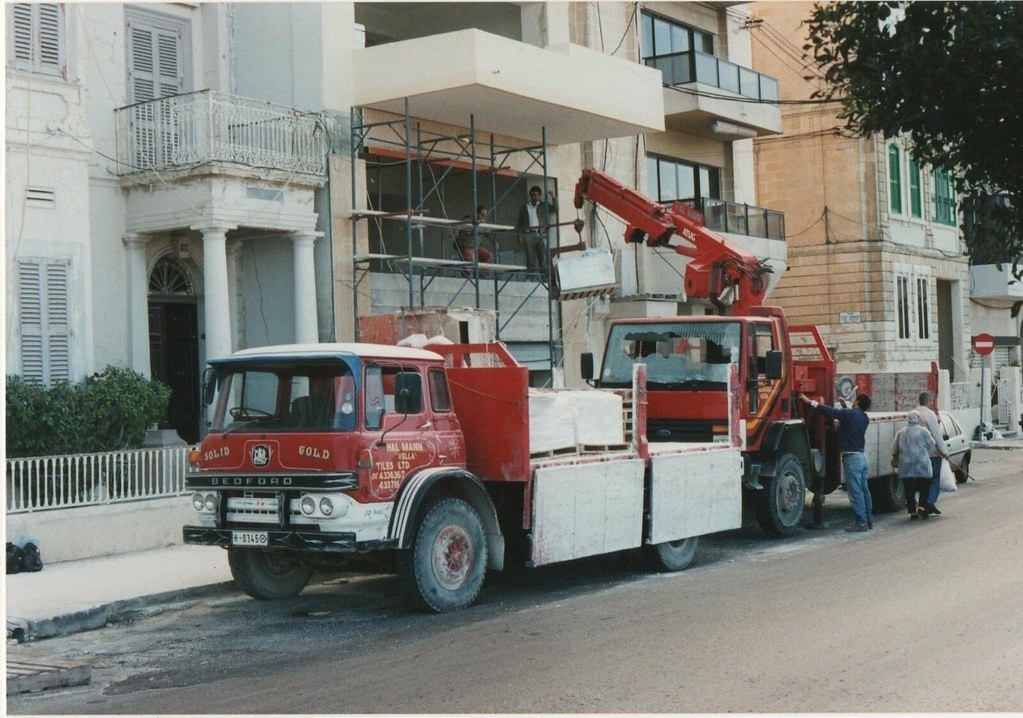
[842,452,860,458]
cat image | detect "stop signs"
[974,332,995,356]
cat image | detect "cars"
[937,408,972,485]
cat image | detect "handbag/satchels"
[940,457,958,492]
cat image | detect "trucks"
[182,336,746,618]
[599,304,942,533]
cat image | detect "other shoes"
[911,513,920,521]
[917,507,929,520]
[844,521,873,532]
[925,501,942,515]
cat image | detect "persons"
[798,392,875,532]
[453,206,494,277]
[915,391,950,514]
[890,411,935,520]
[517,186,559,282]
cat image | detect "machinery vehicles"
[564,168,774,319]
